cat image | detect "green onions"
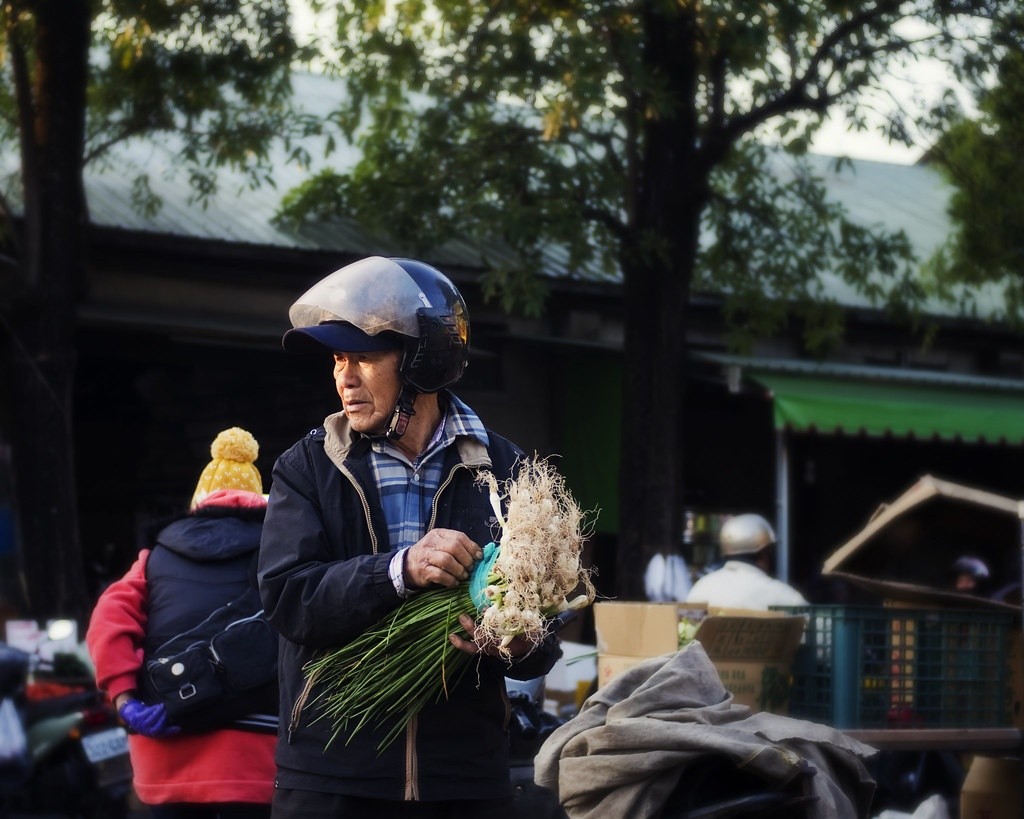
[302,455,596,758]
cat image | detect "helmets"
[952,557,988,577]
[281,257,468,393]
[720,514,775,557]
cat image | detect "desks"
[837,728,1022,752]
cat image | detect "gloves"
[119,699,180,738]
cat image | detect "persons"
[257,256,562,819]
[686,514,810,607]
[83,427,276,818]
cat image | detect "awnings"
[738,369,1024,589]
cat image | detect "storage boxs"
[590,597,804,716]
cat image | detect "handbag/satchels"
[144,589,278,726]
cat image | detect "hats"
[191,427,261,508]
